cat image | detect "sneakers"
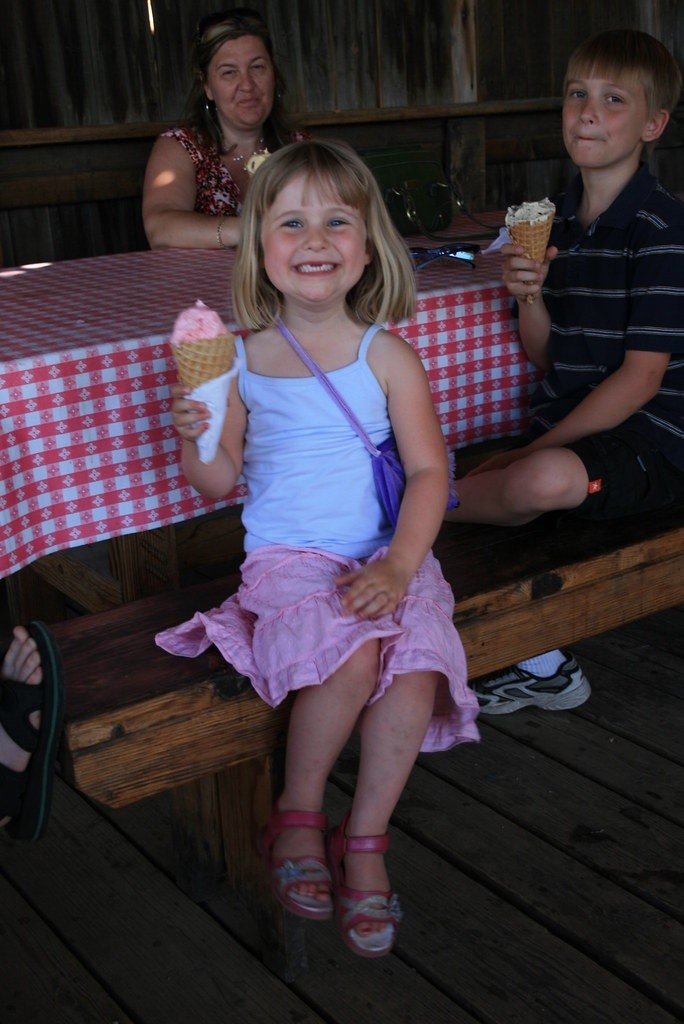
[466,649,592,715]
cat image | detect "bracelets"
[217,217,228,248]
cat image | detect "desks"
[0,208,537,623]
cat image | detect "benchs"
[49,506,683,888]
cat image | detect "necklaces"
[224,146,266,171]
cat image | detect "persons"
[0,621,65,843]
[445,30,684,715]
[155,141,480,958]
[142,9,314,248]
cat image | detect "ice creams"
[505,196,555,305]
[168,298,234,392]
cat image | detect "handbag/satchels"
[369,433,460,532]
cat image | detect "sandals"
[325,809,403,959]
[1,619,65,842]
[260,798,336,921]
